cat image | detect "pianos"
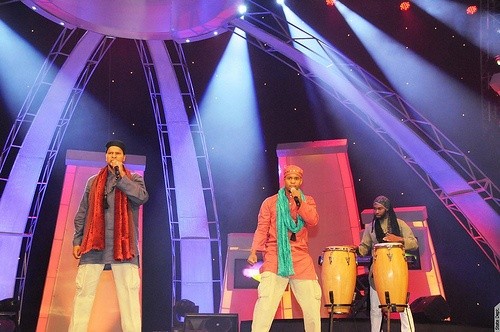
[319,255,417,327]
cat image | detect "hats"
[284,165,304,178]
[105,140,126,154]
[373,195,392,211]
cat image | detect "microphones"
[291,187,300,206]
[115,167,120,179]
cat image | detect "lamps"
[174,298,200,320]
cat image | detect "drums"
[374,242,408,313]
[321,246,357,314]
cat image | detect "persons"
[70,140,149,332]
[350,196,419,332]
[246,165,322,332]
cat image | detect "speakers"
[183,313,240,332]
